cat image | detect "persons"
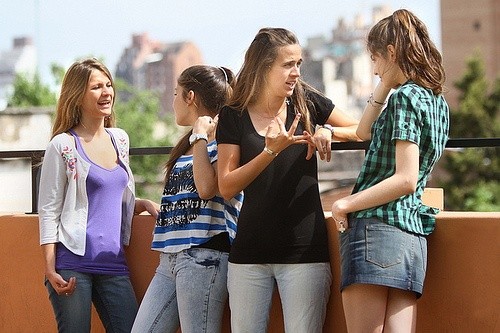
[131,65,244,333]
[331,9,450,333]
[215,27,362,333]
[38,56,161,333]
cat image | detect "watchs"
[189,133,208,146]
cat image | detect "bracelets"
[367,93,386,109]
[332,215,347,233]
[323,123,334,137]
[264,146,278,157]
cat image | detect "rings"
[66,293,68,296]
